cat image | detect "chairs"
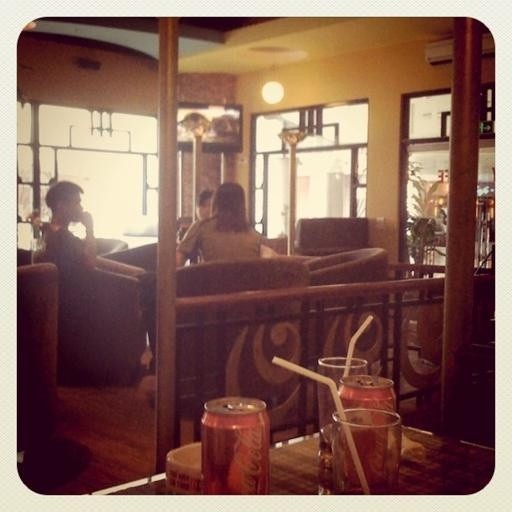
[17,217,388,451]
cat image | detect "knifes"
[425,28,495,65]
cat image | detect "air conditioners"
[425,28,495,65]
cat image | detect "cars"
[318,357,368,469]
[329,407,402,495]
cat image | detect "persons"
[32,178,98,285]
[176,180,262,273]
[176,186,215,268]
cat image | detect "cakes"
[337,374,396,487]
[200,396,271,495]
